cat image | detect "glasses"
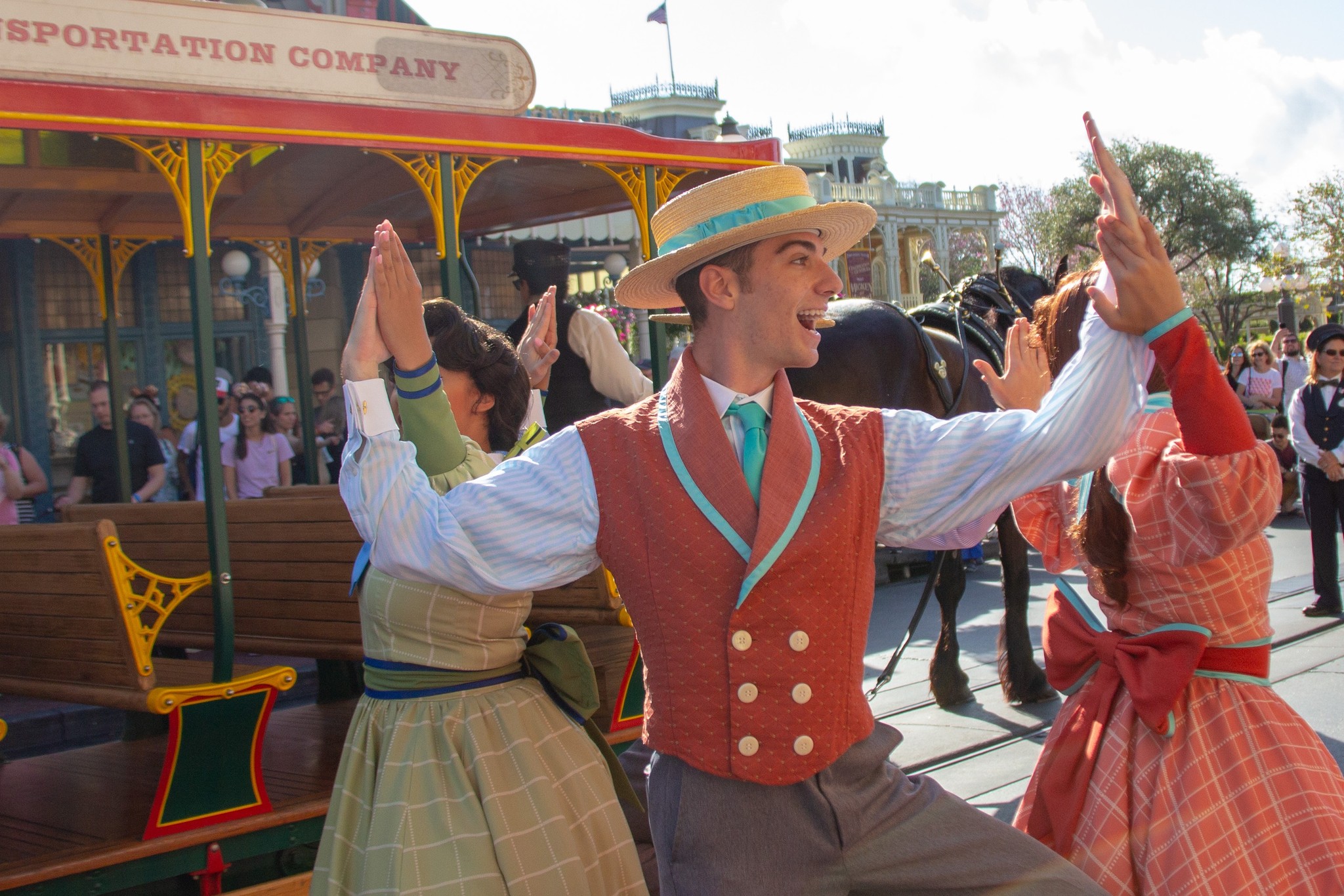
[271,397,295,409]
[1320,349,1344,356]
[314,385,331,397]
[1273,432,1286,439]
[217,397,230,404]
[508,271,523,290]
[1252,351,1266,357]
[1282,338,1297,344]
[238,405,260,413]
[1230,352,1244,358]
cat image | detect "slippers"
[1282,508,1304,517]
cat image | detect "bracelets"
[316,426,320,435]
[133,493,141,503]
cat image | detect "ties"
[722,403,769,510]
[349,540,372,597]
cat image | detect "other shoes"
[1302,604,1342,616]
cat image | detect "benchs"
[64,496,367,662]
[259,485,647,735]
[0,515,300,841]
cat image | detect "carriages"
[1,1,1070,896]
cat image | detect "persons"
[221,394,295,501]
[1265,413,1305,517]
[262,396,339,484]
[1223,344,1251,393]
[314,164,656,896]
[127,398,195,502]
[0,406,48,524]
[247,368,304,453]
[338,112,1158,896]
[1236,340,1283,422]
[312,368,347,484]
[176,377,239,501]
[1270,328,1309,413]
[1287,322,1344,615]
[54,379,167,511]
[975,216,1344,896]
[228,393,240,415]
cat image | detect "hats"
[614,165,877,309]
[1306,323,1344,352]
[214,376,229,398]
[511,239,571,274]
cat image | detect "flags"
[647,2,667,24]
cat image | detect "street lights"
[605,253,651,365]
[1259,240,1308,337]
[220,241,320,430]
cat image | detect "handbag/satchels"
[11,445,54,524]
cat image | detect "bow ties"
[1316,378,1339,388]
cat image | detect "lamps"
[603,255,627,291]
[285,252,327,316]
[217,251,270,319]
